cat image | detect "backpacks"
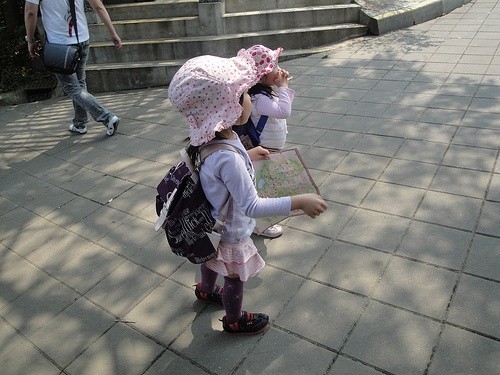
[152,143,242,264]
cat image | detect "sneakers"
[106,116,120,135]
[218,310,270,335]
[192,282,223,300]
[69,123,87,134]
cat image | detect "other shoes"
[252,224,282,238]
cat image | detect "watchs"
[24,35,35,41]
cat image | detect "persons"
[237,44,295,237]
[167,51,329,335]
[25,0,122,136]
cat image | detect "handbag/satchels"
[42,42,81,75]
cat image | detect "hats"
[167,48,258,147]
[247,44,283,78]
